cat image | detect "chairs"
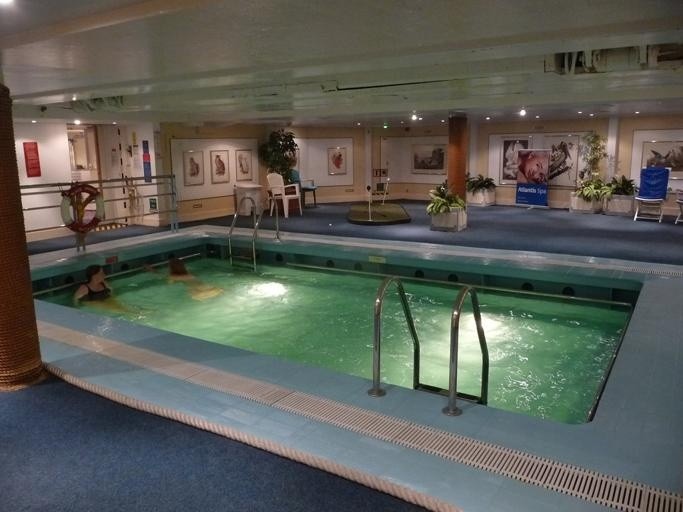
[633,167,672,223]
[300,179,317,207]
[266,173,303,219]
[674,189,683,224]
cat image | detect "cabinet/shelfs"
[235,184,264,217]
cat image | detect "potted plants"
[599,175,638,216]
[258,129,303,215]
[465,174,496,207]
[425,179,467,231]
[568,180,606,214]
[580,135,609,179]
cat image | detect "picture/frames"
[499,136,532,185]
[411,144,448,175]
[235,150,253,181]
[543,135,580,188]
[640,140,683,180]
[183,151,204,185]
[328,147,347,174]
[210,150,230,184]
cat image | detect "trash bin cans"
[234,184,264,217]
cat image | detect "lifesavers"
[61,185,104,233]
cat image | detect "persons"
[144,254,223,301]
[73,264,129,315]
[517,152,549,183]
